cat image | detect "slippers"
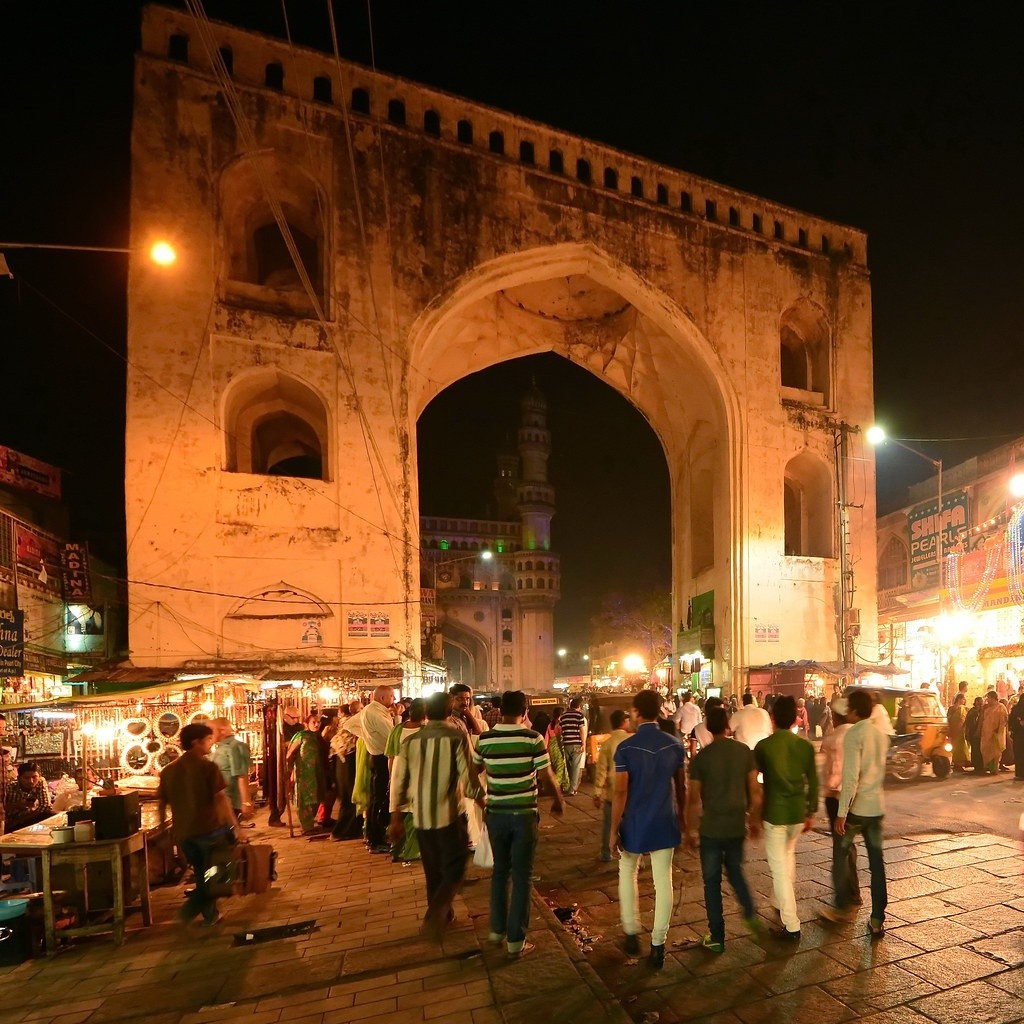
[867,918,885,937]
[488,931,506,943]
[508,941,535,958]
[818,906,858,925]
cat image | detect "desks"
[0,798,173,960]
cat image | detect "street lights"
[865,425,944,710]
[433,552,493,632]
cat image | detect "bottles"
[74,820,95,842]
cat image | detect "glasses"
[286,714,300,719]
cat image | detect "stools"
[14,855,42,892]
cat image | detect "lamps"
[34,705,76,719]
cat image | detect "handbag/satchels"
[397,813,421,861]
[472,821,494,867]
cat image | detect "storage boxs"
[586,734,611,765]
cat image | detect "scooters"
[886,732,925,784]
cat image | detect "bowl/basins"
[0,899,30,920]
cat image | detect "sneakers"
[366,840,391,853]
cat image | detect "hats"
[832,698,849,716]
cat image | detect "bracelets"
[242,802,251,807]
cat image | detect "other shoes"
[184,888,196,895]
[769,927,800,941]
[698,935,724,953]
[569,790,578,796]
[202,913,220,925]
[647,943,666,968]
[268,820,286,827]
[624,934,641,955]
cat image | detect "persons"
[158,724,243,927]
[0,750,53,835]
[268,680,1024,970]
[184,718,252,896]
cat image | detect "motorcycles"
[841,684,952,778]
[585,693,638,785]
